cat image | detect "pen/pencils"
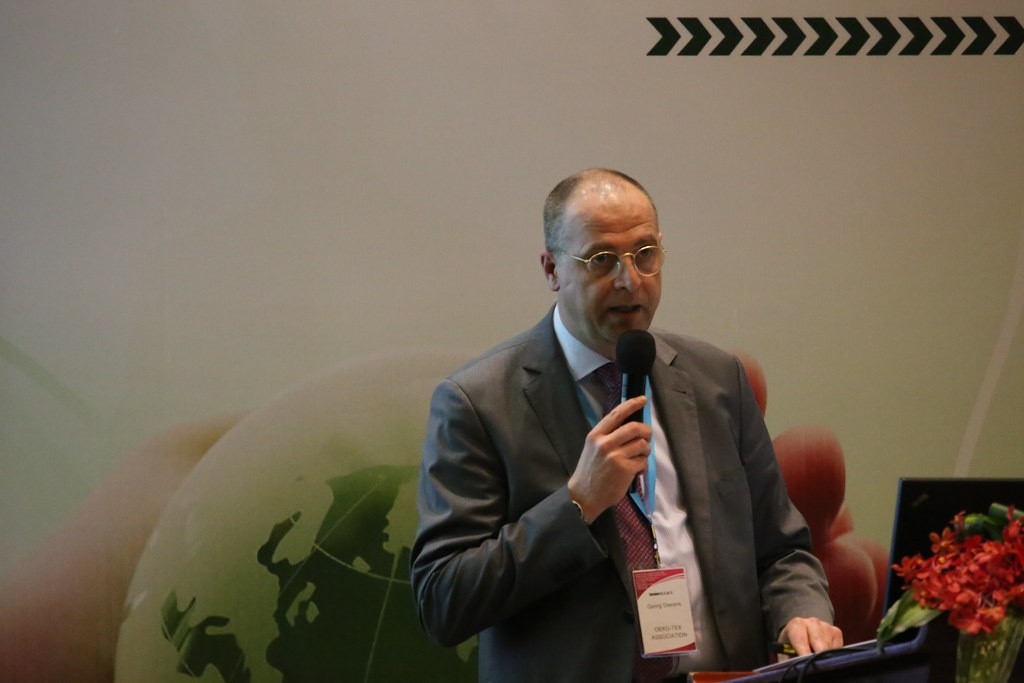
[776,643,798,656]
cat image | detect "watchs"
[557,487,590,532]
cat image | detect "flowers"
[877,505,1024,683]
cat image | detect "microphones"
[617,330,657,493]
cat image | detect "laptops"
[755,477,1024,673]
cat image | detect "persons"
[409,167,844,683]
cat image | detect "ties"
[597,365,675,683]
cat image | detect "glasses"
[548,241,668,282]
